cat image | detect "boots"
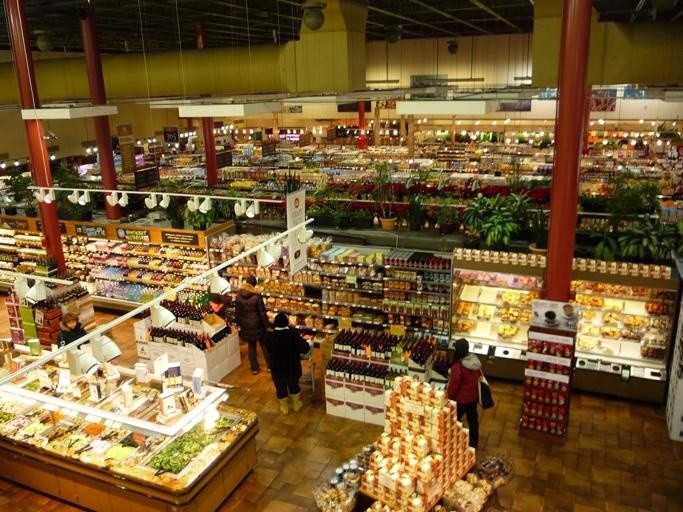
[278,396,289,414]
[288,392,304,412]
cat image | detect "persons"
[420,149,429,159]
[262,313,310,415]
[57,312,91,349]
[389,156,394,169]
[234,275,271,374]
[445,337,482,453]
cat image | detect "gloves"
[307,339,314,348]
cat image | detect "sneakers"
[252,369,272,374]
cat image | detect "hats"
[63,312,77,322]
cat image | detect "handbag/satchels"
[477,368,494,409]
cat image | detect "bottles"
[0,248,215,350]
[325,328,451,393]
